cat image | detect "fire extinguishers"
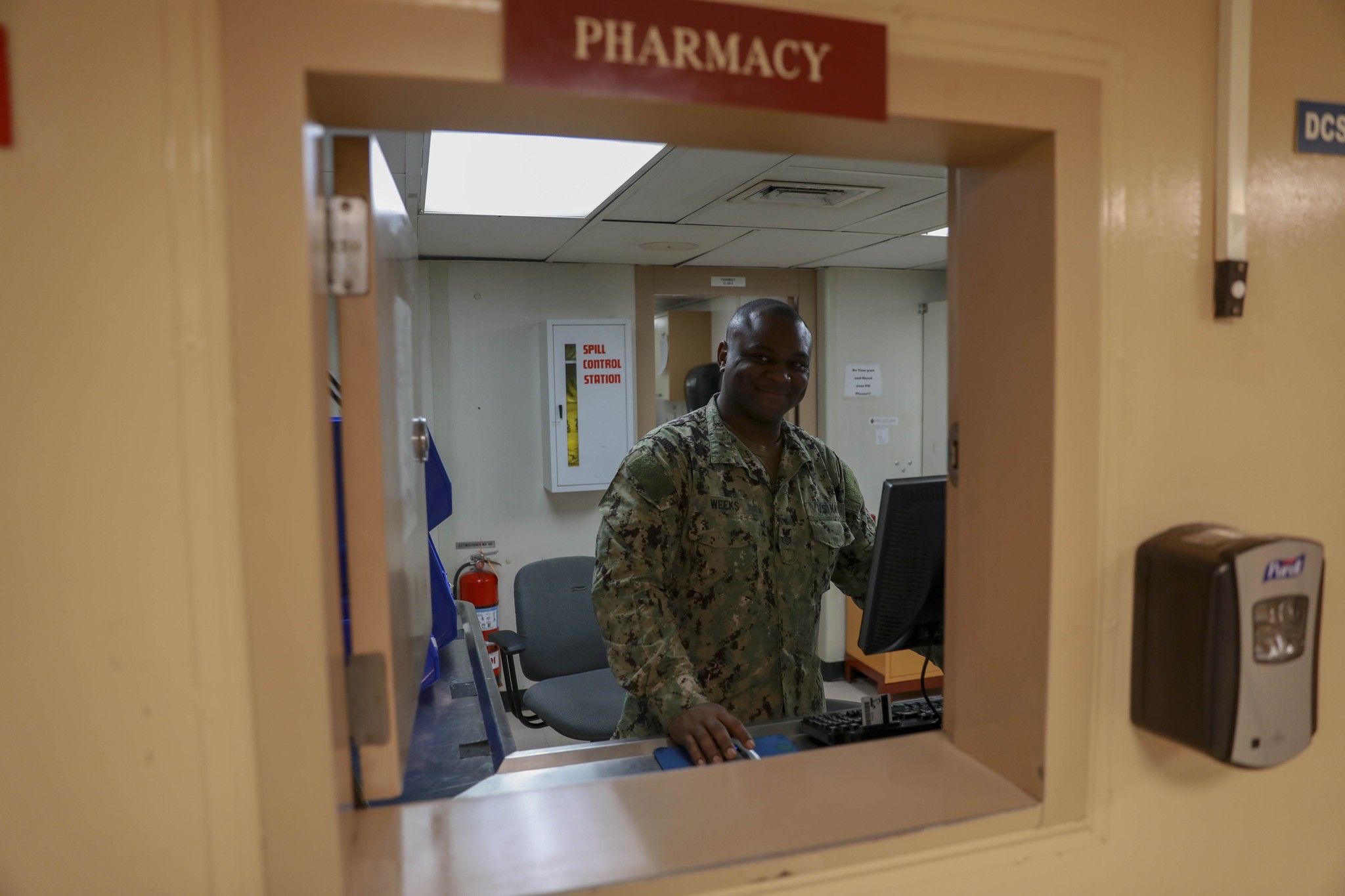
[452,551,503,686]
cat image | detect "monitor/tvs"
[857,472,948,656]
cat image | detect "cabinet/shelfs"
[540,318,635,493]
[845,596,944,702]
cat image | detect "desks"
[349,599,942,808]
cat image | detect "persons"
[591,295,885,773]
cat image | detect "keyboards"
[800,695,943,745]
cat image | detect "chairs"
[487,555,627,743]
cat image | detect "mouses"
[701,736,760,765]
